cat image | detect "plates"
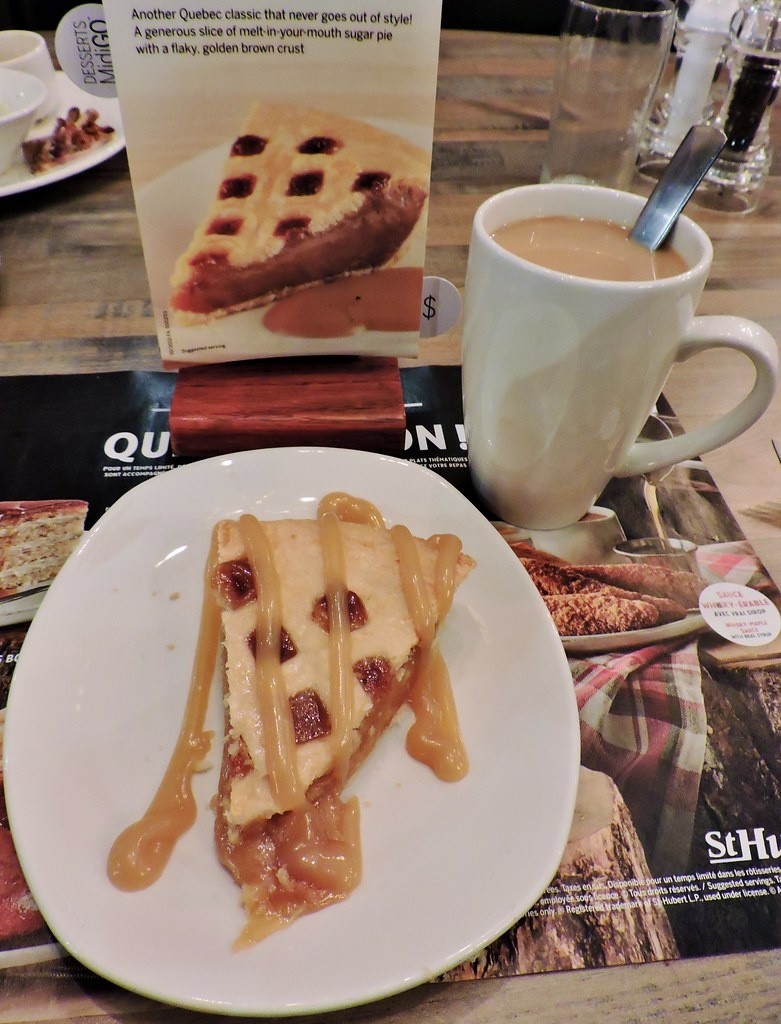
[559,539,757,650]
[0,68,127,199]
[3,446,583,1021]
[134,117,432,363]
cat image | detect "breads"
[509,542,706,637]
[0,499,89,598]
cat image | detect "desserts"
[167,101,430,328]
[107,497,476,949]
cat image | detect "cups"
[0,30,58,123]
[613,538,700,572]
[532,505,628,563]
[463,182,776,532]
[540,0,677,199]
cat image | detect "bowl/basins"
[0,69,47,175]
